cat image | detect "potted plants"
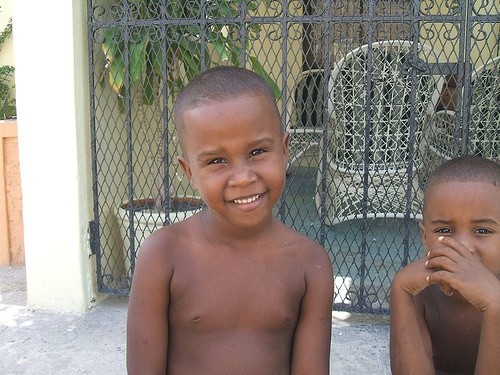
[99,0,283,289]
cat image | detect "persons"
[389,155,500,375]
[126,65,334,375]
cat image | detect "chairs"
[421,56,500,188]
[285,69,333,174]
[315,41,447,226]
